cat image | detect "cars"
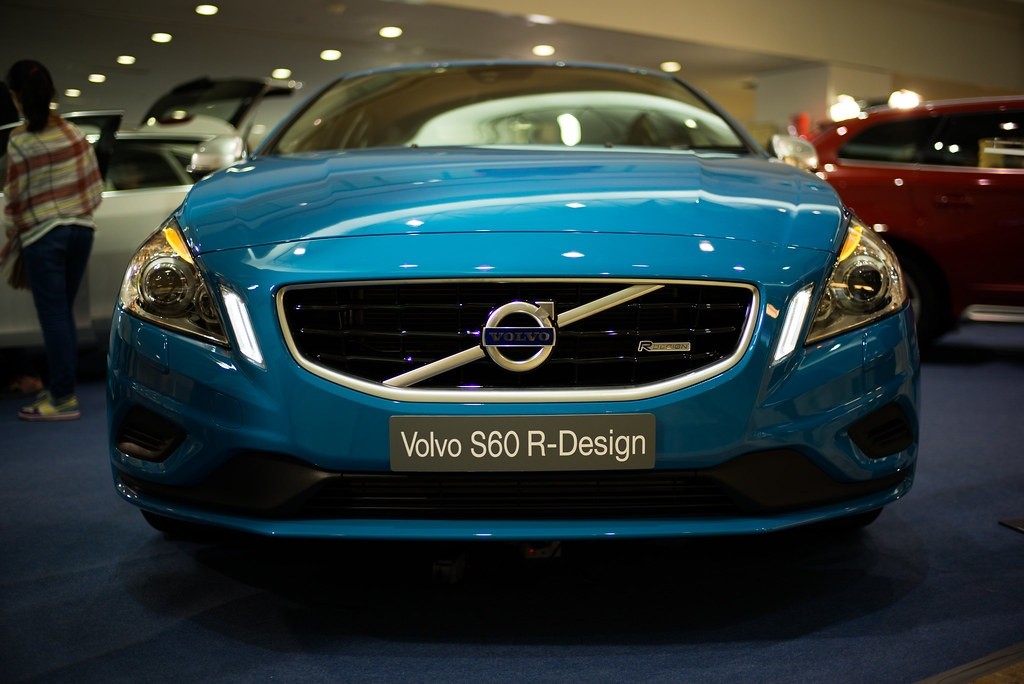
[0,76,295,349]
[809,97,1024,342]
[107,60,919,539]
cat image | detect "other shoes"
[3,238,31,289]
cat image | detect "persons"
[0,60,103,420]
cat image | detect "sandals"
[19,390,82,420]
